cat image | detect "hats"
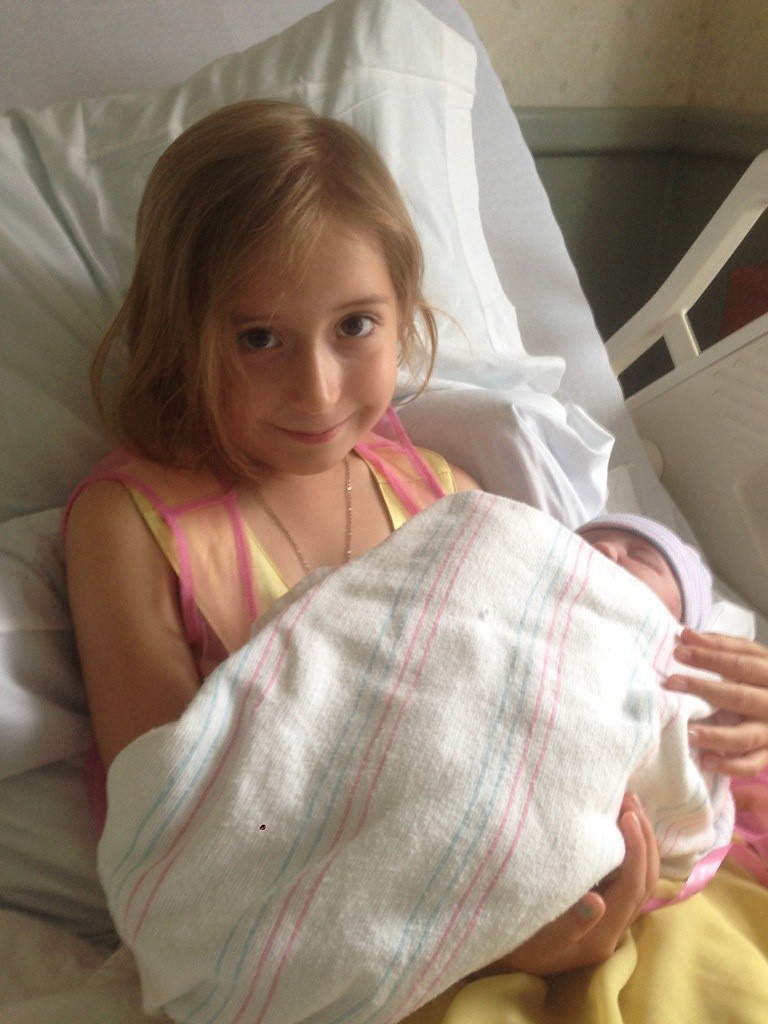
[580,513,713,632]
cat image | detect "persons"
[93,490,714,1024]
[58,101,768,1024]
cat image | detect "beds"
[0,0,768,1024]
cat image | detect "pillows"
[0,0,568,513]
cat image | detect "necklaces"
[249,456,353,577]
